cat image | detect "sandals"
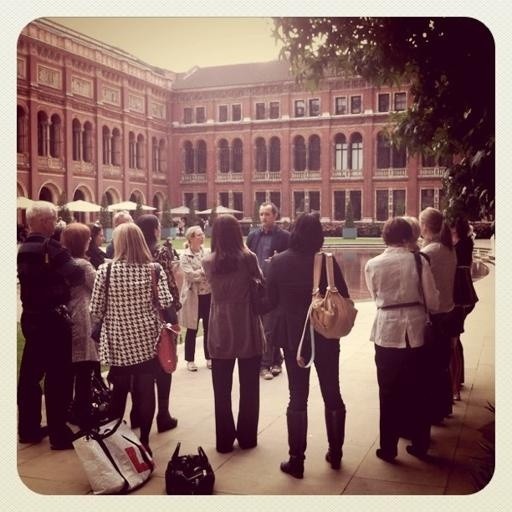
[453,393,459,400]
[206,359,212,369]
[187,362,197,371]
[460,382,464,386]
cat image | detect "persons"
[107,212,133,262]
[246,201,290,380]
[444,209,479,384]
[86,222,106,271]
[59,222,98,432]
[401,206,456,426]
[179,226,212,371]
[89,224,179,458]
[130,215,182,434]
[262,213,349,478]
[364,219,440,462]
[17,202,80,450]
[201,214,265,454]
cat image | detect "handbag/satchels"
[151,263,183,374]
[40,237,72,274]
[414,252,444,342]
[249,276,262,302]
[165,441,215,495]
[471,261,490,282]
[91,263,111,344]
[73,418,154,494]
[295,252,357,369]
[68,370,125,440]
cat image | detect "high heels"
[325,451,341,470]
[130,410,142,429]
[281,458,304,479]
[157,417,178,434]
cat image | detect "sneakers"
[261,368,273,379]
[50,437,76,449]
[407,445,425,459]
[271,365,282,374]
[376,447,394,463]
[239,443,256,449]
[18,427,49,443]
[218,444,232,451]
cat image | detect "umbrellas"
[64,199,104,222]
[156,205,200,216]
[197,205,243,215]
[107,201,157,214]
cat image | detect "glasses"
[93,221,99,237]
